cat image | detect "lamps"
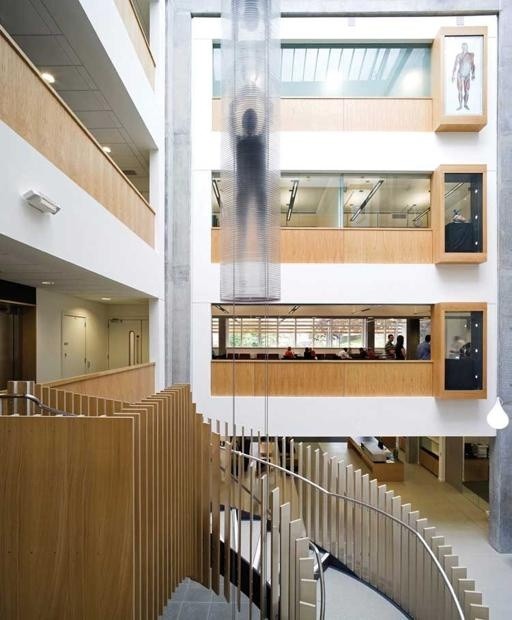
[21,189,61,215]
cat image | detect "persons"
[450,42,476,111]
[230,436,297,476]
[416,333,431,360]
[449,335,467,359]
[285,346,376,360]
[393,334,407,360]
[384,333,395,359]
[225,77,273,298]
[450,208,468,224]
